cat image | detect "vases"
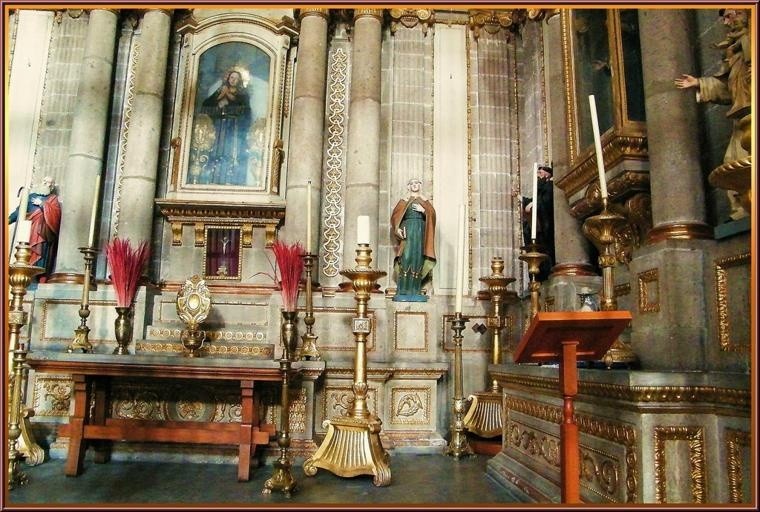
[274,311,299,361]
[112,307,132,355]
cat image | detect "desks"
[26,352,303,482]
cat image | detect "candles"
[589,94,608,201]
[17,219,32,243]
[357,215,372,243]
[87,173,103,245]
[455,204,465,313]
[531,161,538,240]
[306,181,312,252]
[18,187,29,221]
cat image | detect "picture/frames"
[165,12,299,201]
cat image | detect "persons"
[673,9,751,158]
[390,176,437,302]
[511,166,554,281]
[8,176,61,290]
[201,71,250,185]
